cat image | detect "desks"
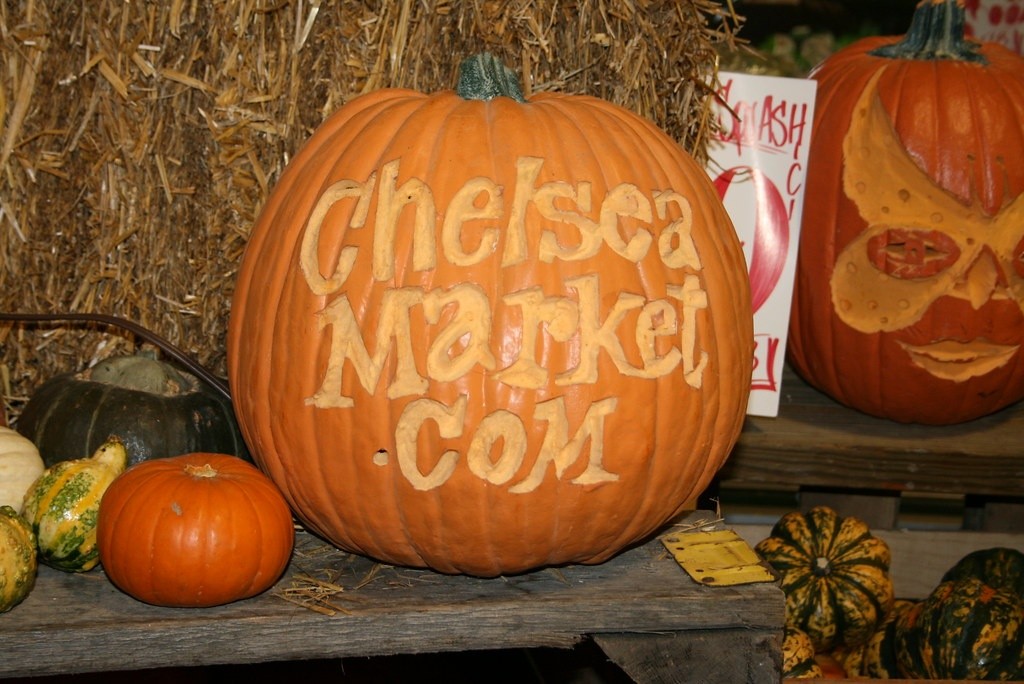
[1,507,787,684]
[700,357,1024,609]
